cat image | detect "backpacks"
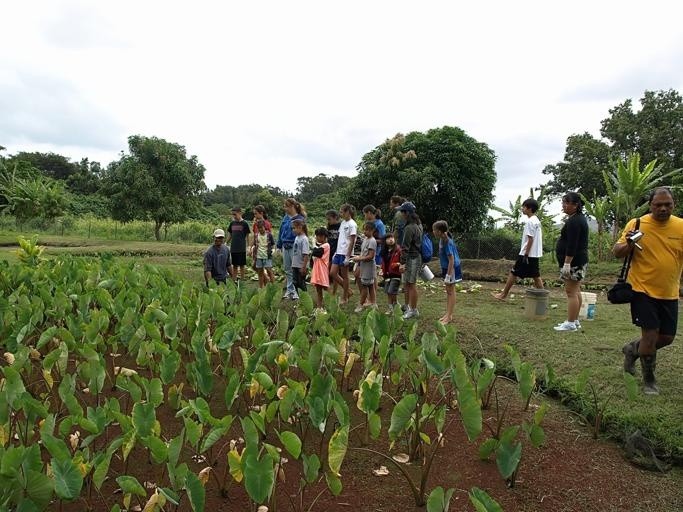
[411,225,434,262]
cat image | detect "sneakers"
[355,298,420,320]
[282,290,299,300]
[314,307,324,316]
[554,320,581,332]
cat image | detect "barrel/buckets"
[525,289,550,321]
[420,264,435,283]
[579,290,597,322]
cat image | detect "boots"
[623,338,660,394]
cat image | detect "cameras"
[626,230,644,250]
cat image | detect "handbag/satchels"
[607,278,634,304]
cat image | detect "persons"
[612,187,683,395]
[201,195,424,321]
[431,219,463,327]
[490,197,544,303]
[552,191,590,332]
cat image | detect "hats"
[394,202,416,211]
[213,229,225,239]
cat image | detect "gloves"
[276,249,280,254]
[444,275,451,286]
[521,254,528,264]
[562,263,571,276]
